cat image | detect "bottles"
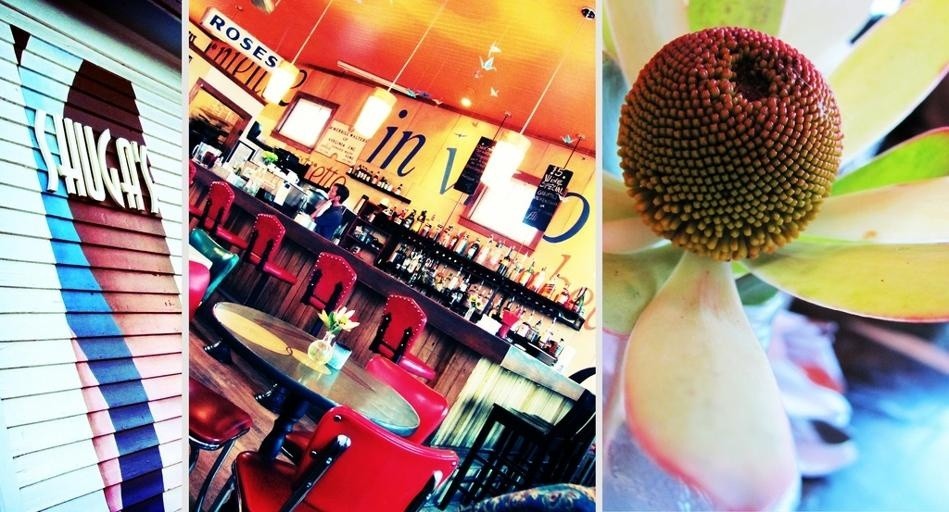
[242,159,270,197]
[346,165,588,366]
[273,180,290,206]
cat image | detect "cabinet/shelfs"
[340,197,584,369]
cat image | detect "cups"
[327,342,352,372]
[191,142,222,169]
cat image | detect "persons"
[311,183,349,242]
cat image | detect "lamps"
[264,1,338,106]
[351,0,454,141]
[481,8,595,193]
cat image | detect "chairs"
[438,391,596,511]
[190,162,459,512]
